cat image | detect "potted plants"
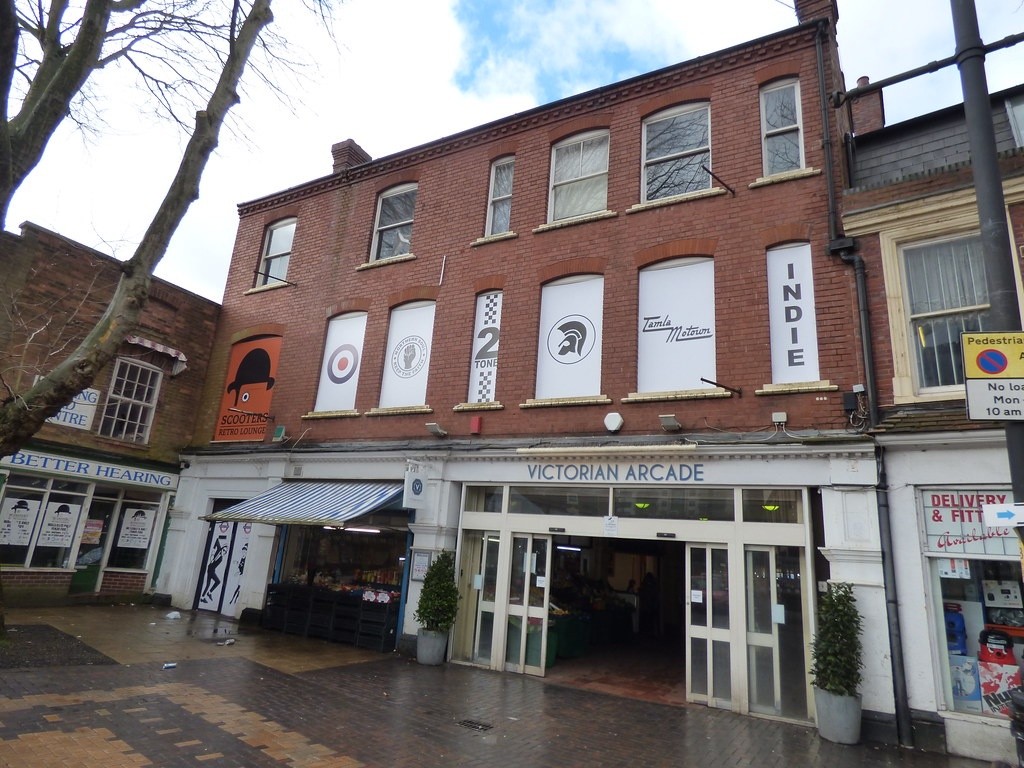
[809,581,865,745]
[413,550,463,666]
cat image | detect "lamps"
[425,423,448,439]
[272,426,288,443]
[659,414,682,432]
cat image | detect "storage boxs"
[240,584,400,653]
[982,580,1023,609]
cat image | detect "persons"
[641,572,659,637]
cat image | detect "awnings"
[198,478,404,528]
